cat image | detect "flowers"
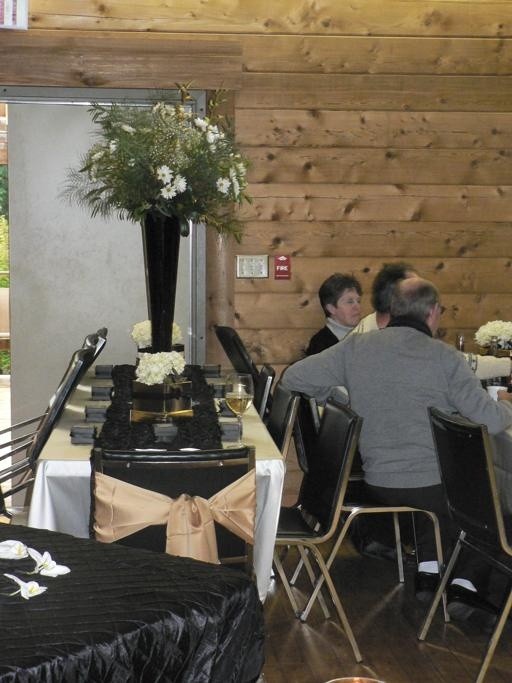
[474,319,511,349]
[54,74,251,244]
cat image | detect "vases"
[140,213,181,353]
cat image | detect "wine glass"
[223,372,255,450]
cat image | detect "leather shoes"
[415,572,448,623]
[361,538,409,566]
[446,583,499,627]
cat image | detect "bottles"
[456,332,465,351]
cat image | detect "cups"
[485,386,509,404]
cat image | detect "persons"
[336,263,512,566]
[306,272,363,356]
[281,277,512,628]
